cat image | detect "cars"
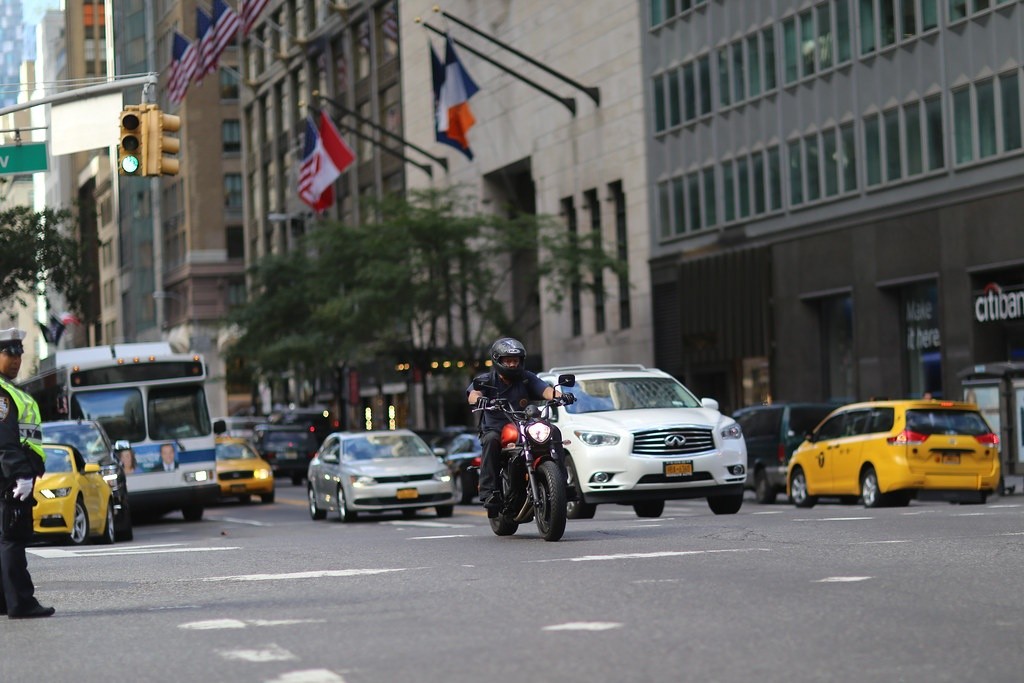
[438,430,483,504]
[305,428,458,524]
[784,396,1001,509]
[212,436,276,505]
[251,423,317,488]
[31,442,116,546]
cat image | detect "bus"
[10,338,228,526]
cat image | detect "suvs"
[38,418,135,542]
[729,400,848,505]
[526,362,748,518]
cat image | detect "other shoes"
[486,494,501,518]
[8,601,55,618]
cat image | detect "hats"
[0,328,26,350]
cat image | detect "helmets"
[489,339,527,381]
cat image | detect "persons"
[0,328,55,617]
[465,338,574,519]
[152,444,178,471]
[118,448,143,473]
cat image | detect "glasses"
[0,347,23,356]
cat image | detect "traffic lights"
[118,110,142,177]
[147,109,181,178]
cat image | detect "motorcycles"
[471,371,577,542]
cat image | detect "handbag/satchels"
[2,491,36,540]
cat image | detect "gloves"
[12,477,33,502]
[560,393,575,406]
[476,396,490,409]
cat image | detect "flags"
[429,32,479,160]
[298,109,355,213]
[40,311,80,346]
[166,0,270,106]
